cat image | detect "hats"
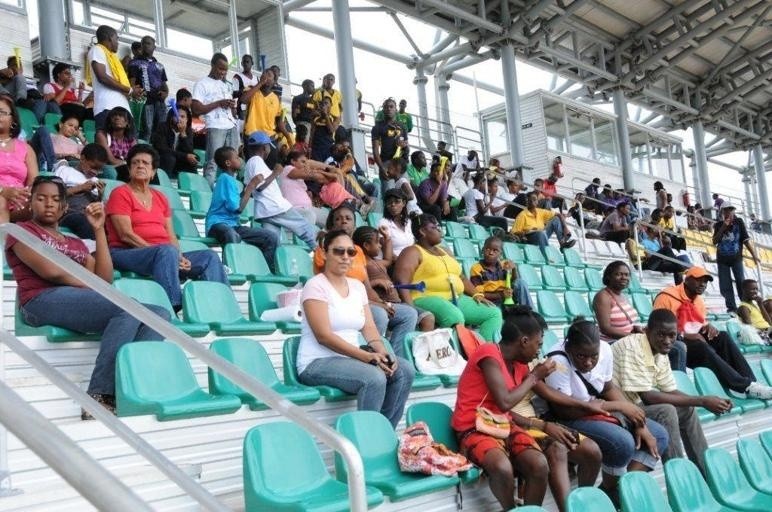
[383,188,409,200]
[685,266,714,283]
[719,201,737,211]
[247,130,276,149]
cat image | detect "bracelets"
[128,87,133,95]
[472,293,485,298]
[528,374,536,385]
[82,139,86,145]
[367,338,384,347]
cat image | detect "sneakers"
[743,383,772,402]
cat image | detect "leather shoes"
[560,238,576,253]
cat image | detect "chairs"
[177,240,247,286]
[112,340,243,422]
[435,171,461,206]
[154,168,191,197]
[686,240,692,249]
[653,371,716,424]
[188,148,208,168]
[620,240,630,255]
[728,323,761,355]
[664,458,744,512]
[222,243,299,288]
[39,168,57,183]
[564,292,596,323]
[206,336,321,413]
[754,340,769,355]
[468,224,494,243]
[403,331,465,389]
[720,330,742,357]
[1,231,121,282]
[100,178,126,201]
[82,118,103,147]
[565,486,618,512]
[405,401,484,487]
[758,430,772,460]
[583,237,594,253]
[583,267,608,293]
[698,311,716,323]
[111,277,209,338]
[487,226,506,238]
[282,336,360,403]
[450,178,474,202]
[541,265,567,293]
[562,266,590,292]
[695,231,702,242]
[626,271,650,294]
[672,247,679,259]
[694,244,703,256]
[13,285,106,343]
[537,290,578,325]
[708,248,716,260]
[272,245,316,285]
[247,282,304,335]
[501,242,527,264]
[707,261,720,274]
[467,180,483,196]
[178,171,220,195]
[704,233,713,246]
[591,239,613,257]
[62,159,121,181]
[446,221,480,244]
[735,437,771,495]
[693,367,743,419]
[710,279,726,296]
[745,361,771,407]
[761,359,772,389]
[703,447,759,512]
[360,337,442,391]
[495,185,506,202]
[681,228,695,241]
[616,471,673,511]
[13,107,40,140]
[588,291,600,313]
[563,225,579,242]
[587,264,604,271]
[172,209,223,247]
[517,264,544,291]
[242,419,385,512]
[631,293,654,324]
[44,113,78,147]
[363,209,389,232]
[333,410,462,504]
[697,279,715,298]
[714,307,730,321]
[724,384,766,413]
[544,246,566,267]
[648,268,671,290]
[189,190,249,223]
[540,331,558,359]
[680,248,690,266]
[522,245,546,265]
[453,238,486,261]
[180,279,277,337]
[692,247,705,269]
[148,185,208,220]
[562,247,586,269]
[737,230,772,279]
[605,240,628,259]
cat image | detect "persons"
[158,105,200,179]
[543,173,568,216]
[43,64,94,121]
[593,260,643,345]
[388,158,423,216]
[511,192,576,253]
[416,164,458,226]
[18,126,56,173]
[243,132,320,249]
[641,209,673,248]
[397,99,413,133]
[355,78,362,112]
[295,230,415,432]
[5,176,172,420]
[313,206,418,359]
[458,175,508,233]
[712,201,761,318]
[275,115,295,148]
[129,36,169,140]
[232,55,261,143]
[240,69,293,149]
[394,214,503,344]
[270,65,283,104]
[470,236,534,311]
[0,92,39,224]
[85,25,144,130]
[582,178,602,215]
[0,68,35,108]
[712,194,724,209]
[748,214,763,233]
[456,151,481,184]
[736,279,772,345]
[568,193,604,229]
[531,179,552,210]
[374,106,385,122]
[667,193,674,209]
[506,390,603,512]
[654,181,668,209]
[452,305,610,512]
[54,144,107,239]
[659,206,686,249]
[292,124,311,156]
[599,184,616,217]
[279,151,330,227]
[543,316,670,509]
[205,146,278,273]
[95,106,136,164]
[624,223,688,286]
[485,178,526,219]
[353,226,435,331]
[311,97,340,161]
[132,42,142,56]
[168,88,191,125]
[191,53,239,187]
[642,222,691,263]
[599,202,630,243]
[653,267,772,399]
[688,201,708,233]
[320,140,375,214]
[192,115,206,148]
[312,74,343,119]
[7,55,63,122]
[430,141,452,171]
[105,144,232,314]
[407,151,430,187]
[488,158,506,185]
[50,114,89,161]
[611,308,734,480]
[378,189,415,259]
[441,156,465,220]
[292,79,319,142]
[372,100,409,193]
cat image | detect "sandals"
[82,394,118,422]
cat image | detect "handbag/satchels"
[472,344,514,440]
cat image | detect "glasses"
[324,246,359,258]
[30,175,67,195]
[383,199,405,206]
[420,224,441,232]
[0,109,14,119]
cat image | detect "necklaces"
[0,136,11,146]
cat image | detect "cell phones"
[382,354,395,368]
